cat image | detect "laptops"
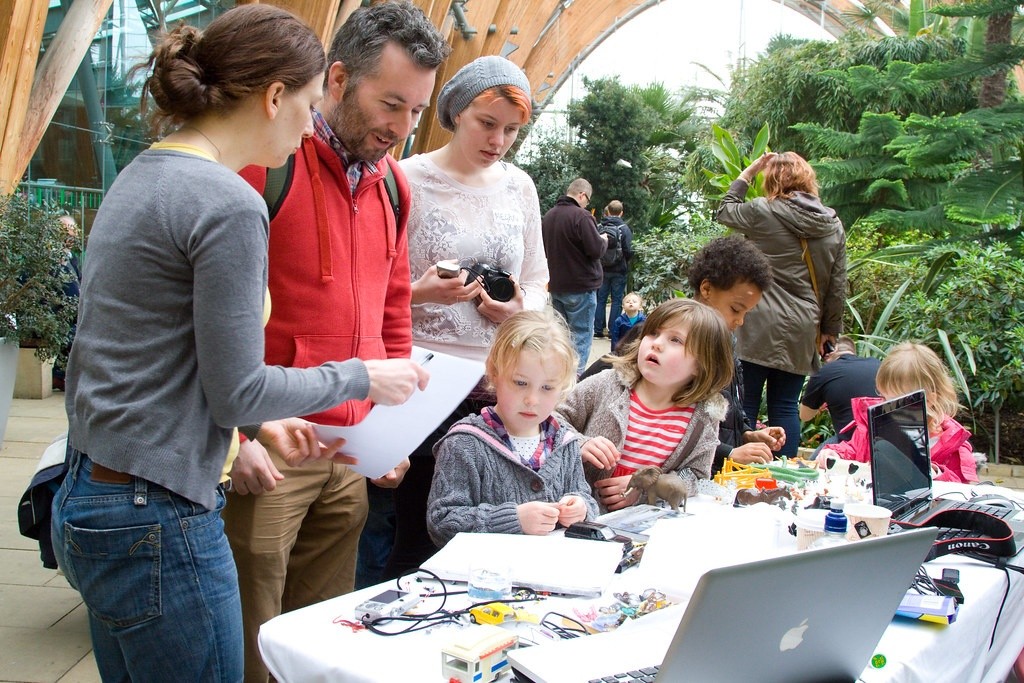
[506,522,940,683]
[867,388,1021,558]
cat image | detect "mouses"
[969,493,1015,508]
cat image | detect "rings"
[456,296,460,304]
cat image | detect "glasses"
[583,193,591,206]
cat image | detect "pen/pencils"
[615,547,646,573]
[417,354,435,369]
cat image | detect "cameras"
[464,261,516,303]
[352,589,421,625]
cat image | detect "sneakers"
[593,332,605,340]
[603,333,612,340]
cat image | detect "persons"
[427,310,600,549]
[16,212,87,394]
[355,55,550,592]
[541,179,650,385]
[800,335,979,484]
[715,151,846,463]
[577,236,787,482]
[52,3,430,683]
[552,299,736,513]
[220,0,454,683]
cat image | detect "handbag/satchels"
[17,435,74,568]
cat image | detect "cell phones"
[435,259,460,280]
[819,340,834,362]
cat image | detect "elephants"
[621,466,687,515]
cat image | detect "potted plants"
[0,190,83,441]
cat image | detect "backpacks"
[598,223,627,272]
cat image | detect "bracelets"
[739,172,752,184]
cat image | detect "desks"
[259,458,1024,683]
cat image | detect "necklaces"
[182,123,227,167]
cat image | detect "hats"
[437,55,535,134]
